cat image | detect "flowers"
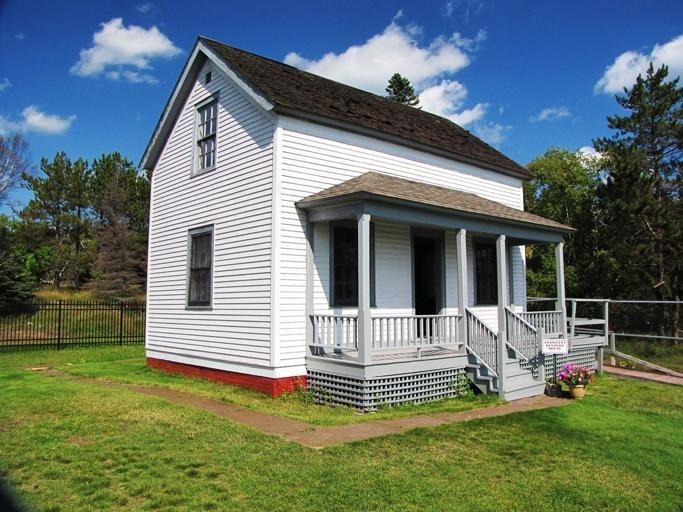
[556,362,594,385]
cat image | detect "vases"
[569,385,587,400]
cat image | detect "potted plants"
[545,377,562,397]
